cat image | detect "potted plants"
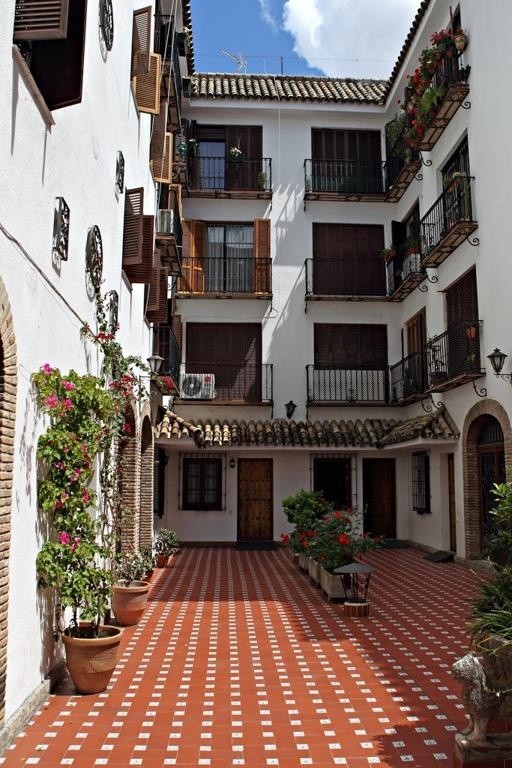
[154,527,179,569]
[460,557,512,721]
[402,237,421,258]
[376,242,397,260]
[108,549,155,629]
[425,335,449,385]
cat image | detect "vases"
[60,621,123,694]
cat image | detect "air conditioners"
[178,371,216,401]
[156,207,175,236]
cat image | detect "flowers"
[229,146,243,160]
[279,487,375,597]
[27,363,124,633]
[400,25,471,151]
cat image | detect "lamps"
[147,350,165,375]
[284,400,298,419]
[485,346,512,384]
[228,456,236,469]
